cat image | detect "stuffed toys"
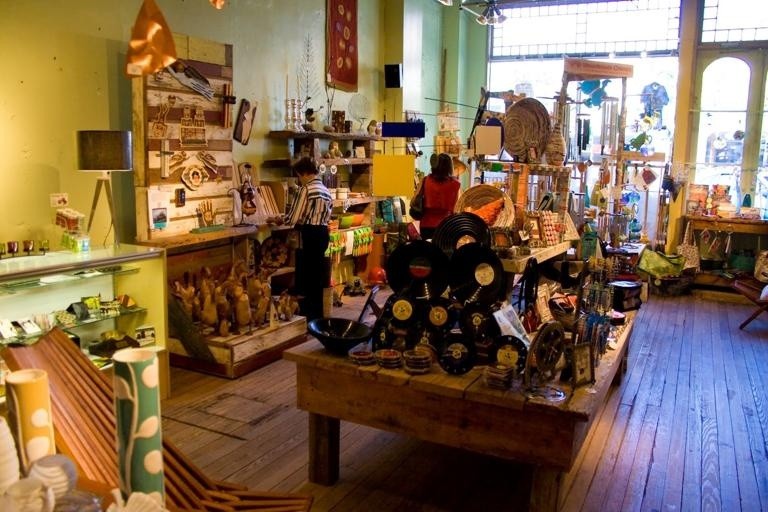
[323,142,343,159]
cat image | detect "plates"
[384,294,526,377]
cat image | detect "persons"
[411,153,461,241]
[265,156,332,318]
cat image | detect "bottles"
[55,206,90,252]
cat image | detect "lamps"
[476,0,506,25]
[75,130,133,247]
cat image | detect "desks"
[285,302,641,512]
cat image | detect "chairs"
[733,280,768,330]
[0,328,315,512]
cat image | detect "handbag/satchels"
[409,192,429,222]
[676,240,702,276]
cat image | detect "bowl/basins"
[337,214,365,229]
[307,318,374,355]
[384,214,507,305]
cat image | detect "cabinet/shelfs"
[0,243,172,428]
[270,130,376,300]
[681,211,768,302]
